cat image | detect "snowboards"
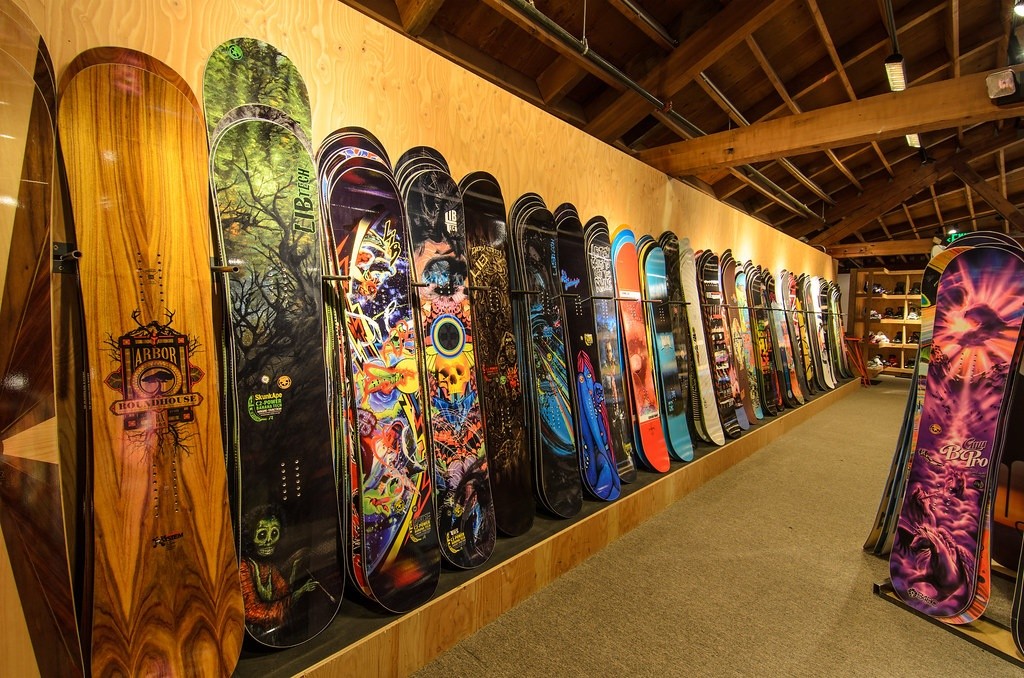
[864,227,1024,626]
[1,0,855,678]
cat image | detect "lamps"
[905,132,922,150]
[884,53,909,92]
[1013,0,1024,17]
[985,68,1023,108]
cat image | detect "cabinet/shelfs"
[849,268,926,377]
